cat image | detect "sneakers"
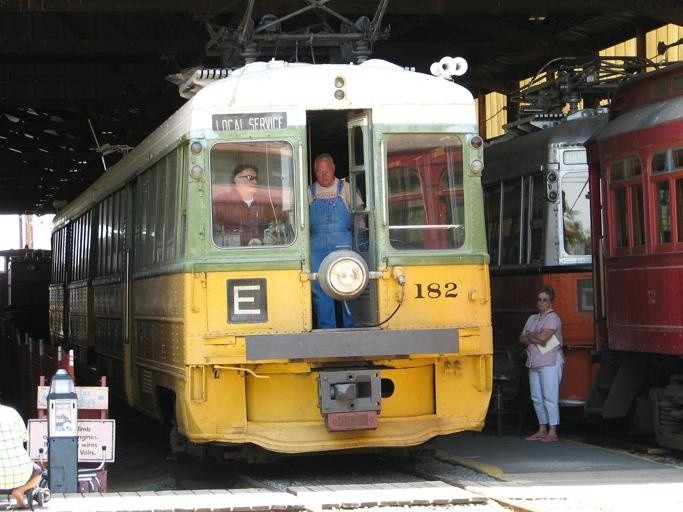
[525,433,558,441]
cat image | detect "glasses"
[537,298,548,302]
[236,175,259,181]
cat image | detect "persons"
[518,285,565,441]
[0,405,42,508]
[308,153,365,329]
[213,165,290,245]
